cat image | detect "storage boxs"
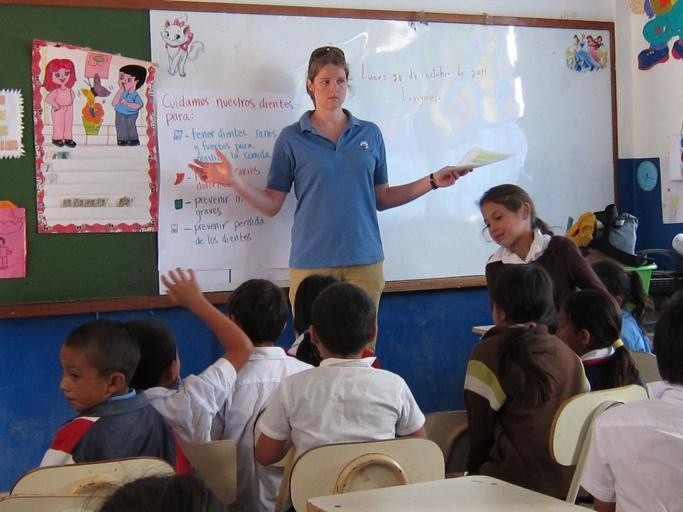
[621,263,657,323]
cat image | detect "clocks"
[618,157,666,264]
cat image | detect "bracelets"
[429,172,438,191]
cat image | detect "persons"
[476,182,609,336]
[38,267,428,512]
[446,262,683,511]
[183,45,475,353]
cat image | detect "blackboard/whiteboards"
[1,0,620,318]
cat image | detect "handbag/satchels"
[584,203,640,268]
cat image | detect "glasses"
[309,46,345,67]
